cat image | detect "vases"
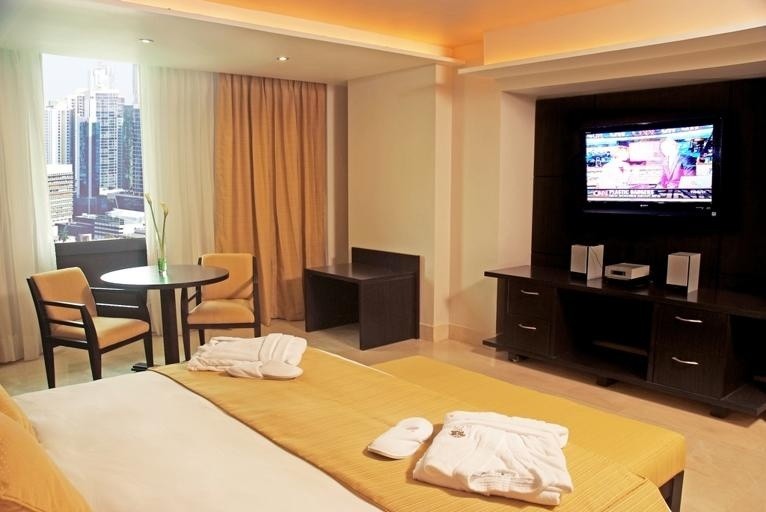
[154,231,167,272]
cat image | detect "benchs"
[372,355,687,512]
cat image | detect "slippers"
[227,361,304,380]
[368,416,434,460]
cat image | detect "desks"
[302,263,418,351]
[100,263,230,373]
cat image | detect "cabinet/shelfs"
[481,264,764,421]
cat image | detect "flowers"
[144,192,169,259]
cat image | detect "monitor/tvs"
[578,113,724,222]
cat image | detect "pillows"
[0,385,96,512]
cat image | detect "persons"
[595,146,632,189]
[660,137,695,188]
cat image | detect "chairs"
[180,252,261,361]
[25,265,155,388]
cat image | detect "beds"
[0,338,671,512]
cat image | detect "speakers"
[666,251,702,292]
[570,244,604,280]
